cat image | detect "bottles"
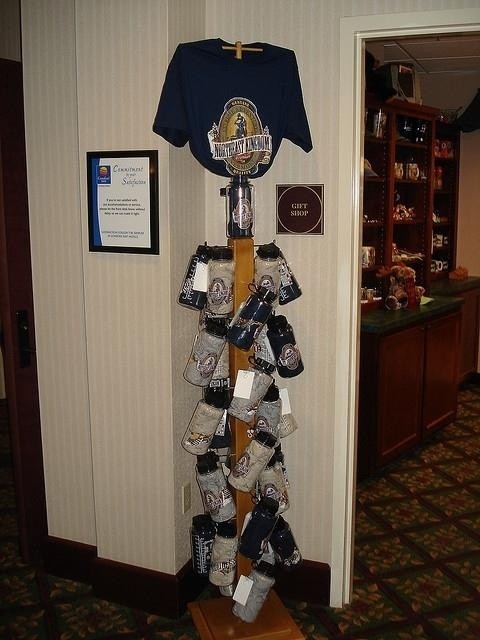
[226,174,255,241]
[435,166,443,191]
[180,240,304,626]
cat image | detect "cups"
[434,234,444,247]
[366,288,374,303]
[373,113,387,138]
[431,259,449,273]
[362,246,376,269]
[395,161,420,181]
